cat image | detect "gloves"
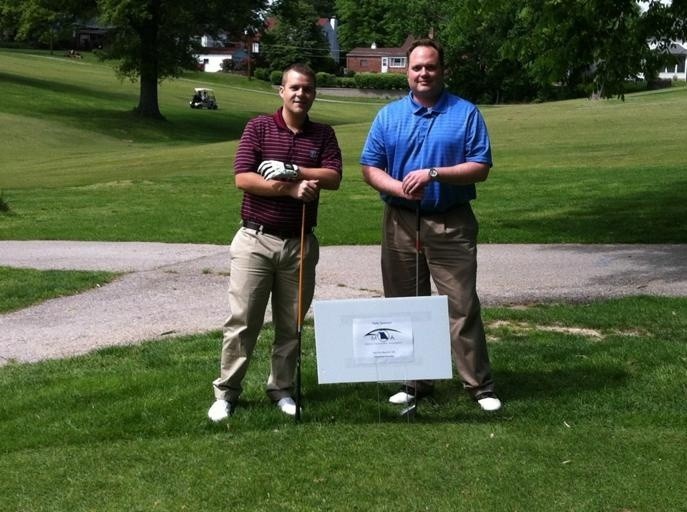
[257,159,299,181]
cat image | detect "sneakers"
[208,400,231,422]
[277,397,302,414]
[389,391,416,404]
[477,397,501,411]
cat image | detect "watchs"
[427,167,438,180]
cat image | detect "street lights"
[247,35,259,80]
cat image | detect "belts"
[397,205,440,217]
[245,222,301,238]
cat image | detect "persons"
[360,37,504,413]
[204,61,342,424]
